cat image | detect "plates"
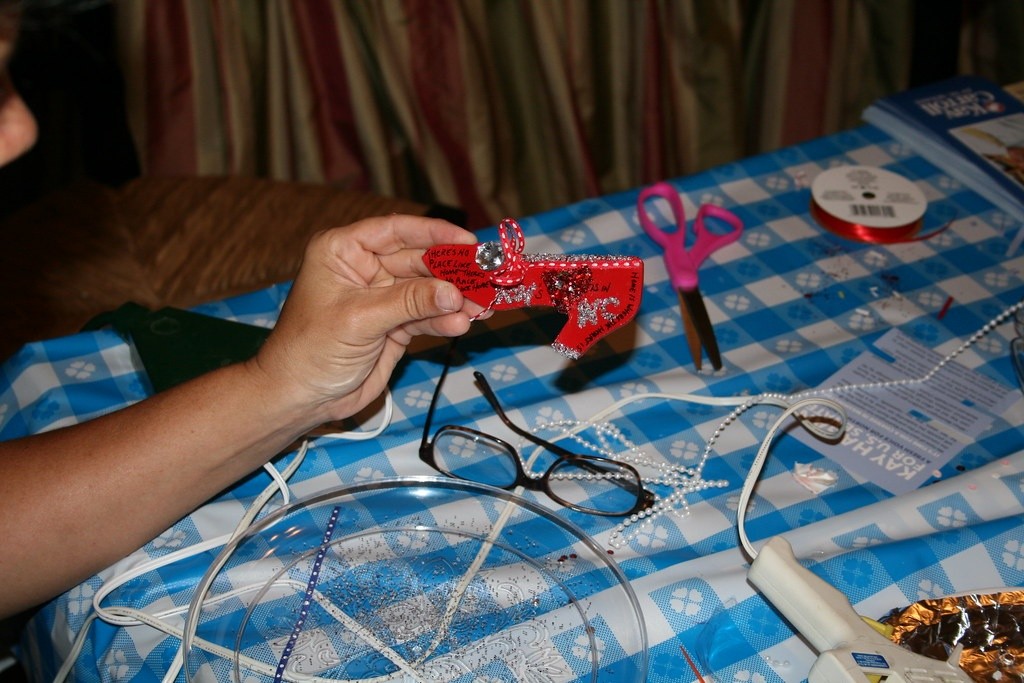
[179,474,650,683]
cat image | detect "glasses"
[419,338,654,517]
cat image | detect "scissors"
[635,181,743,371]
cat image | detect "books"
[861,74,1023,223]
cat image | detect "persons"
[0,1,496,683]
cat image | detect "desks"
[0,123,1024,683]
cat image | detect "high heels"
[421,217,644,360]
